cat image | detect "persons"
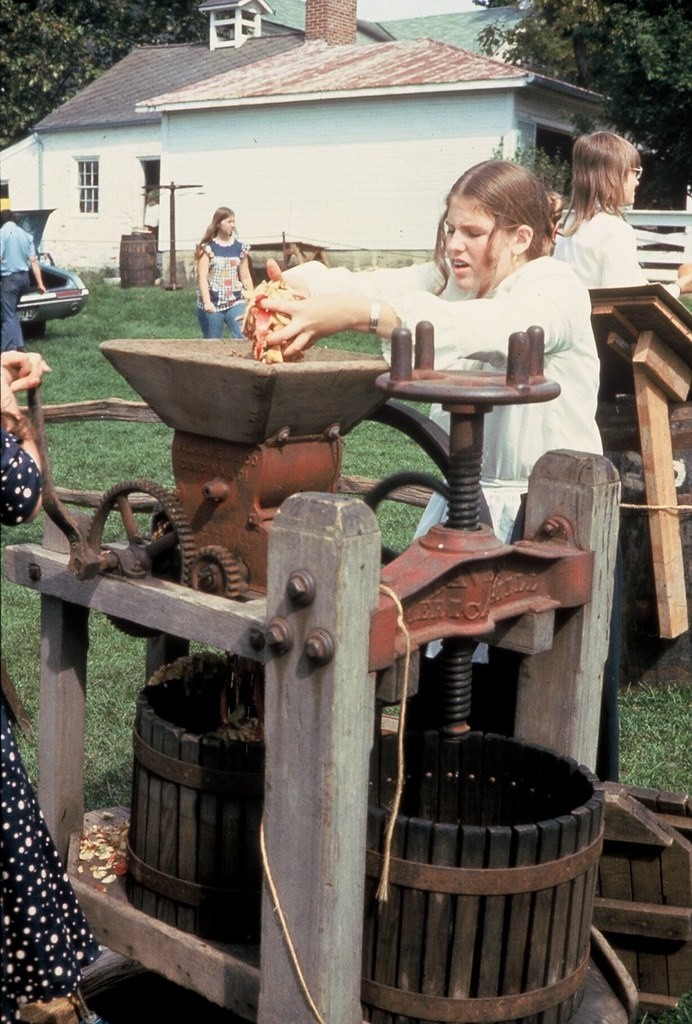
[1,209,46,354]
[195,206,254,340]
[249,159,621,782]
[0,350,103,1024]
[552,132,692,490]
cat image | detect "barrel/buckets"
[360,732,605,1024]
[127,652,262,946]
[120,235,158,288]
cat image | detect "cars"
[11,209,89,340]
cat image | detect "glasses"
[631,166,642,179]
